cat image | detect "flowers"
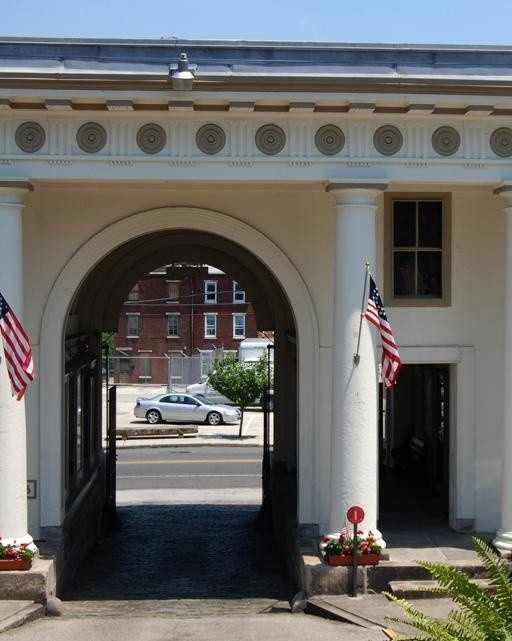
[0,535,36,561]
[318,527,382,556]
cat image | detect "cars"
[133,391,241,425]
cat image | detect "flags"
[1,294,37,403]
[358,272,404,391]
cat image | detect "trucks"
[184,336,274,413]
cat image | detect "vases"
[0,559,32,570]
[326,553,382,565]
